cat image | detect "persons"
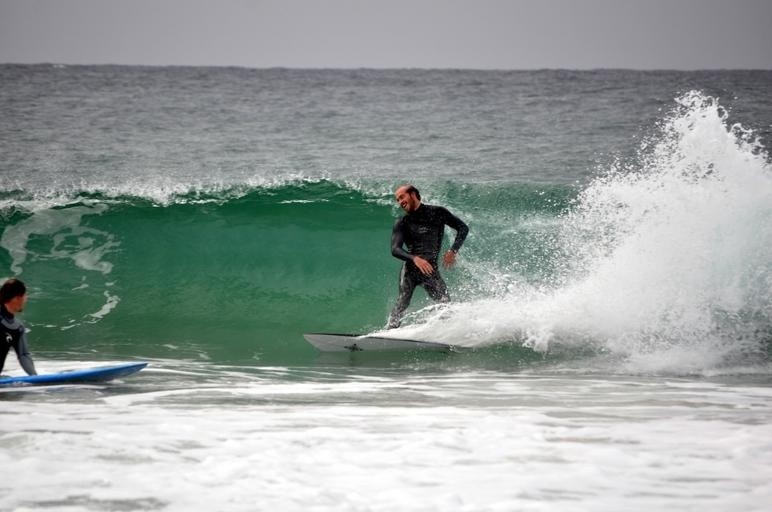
[0,279,37,376]
[383,184,467,330]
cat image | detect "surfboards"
[301,330,453,353]
[0,359,147,388]
